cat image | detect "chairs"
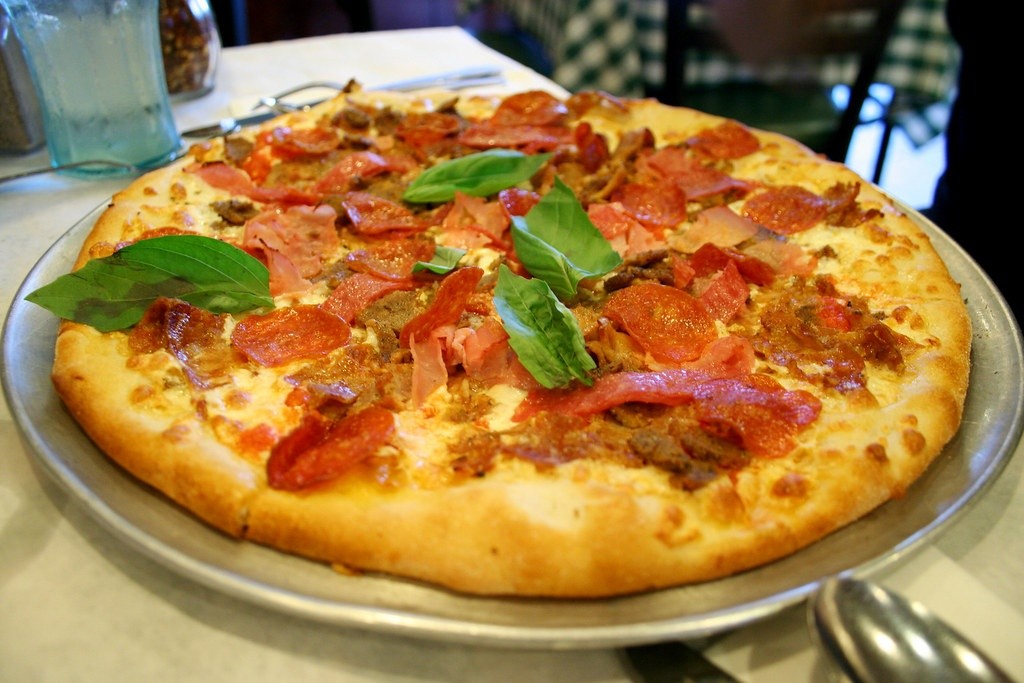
[648,0,893,162]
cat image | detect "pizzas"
[50,90,975,597]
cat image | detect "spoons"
[806,576,1016,683]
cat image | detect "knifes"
[182,66,507,137]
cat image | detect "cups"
[0,0,183,181]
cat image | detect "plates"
[0,185,1024,651]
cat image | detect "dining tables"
[0,26,1024,683]
